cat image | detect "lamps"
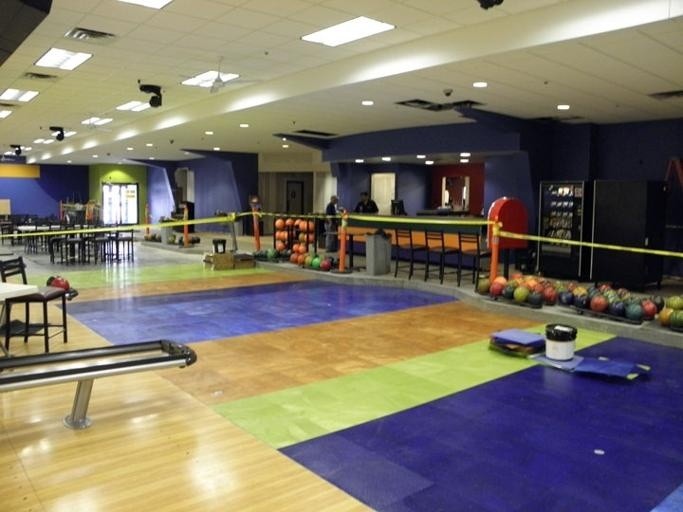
[139,84,163,107]
[49,126,63,141]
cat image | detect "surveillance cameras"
[56,133,65,140]
[169,140,174,144]
[443,89,453,96]
[16,149,21,156]
[150,96,160,108]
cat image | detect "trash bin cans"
[366,229,392,276]
[545,324,577,361]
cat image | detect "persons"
[324,196,338,252]
[354,192,379,212]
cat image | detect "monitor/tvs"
[391,199,407,216]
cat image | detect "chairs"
[392,225,491,293]
[16,221,135,265]
[0,256,67,355]
